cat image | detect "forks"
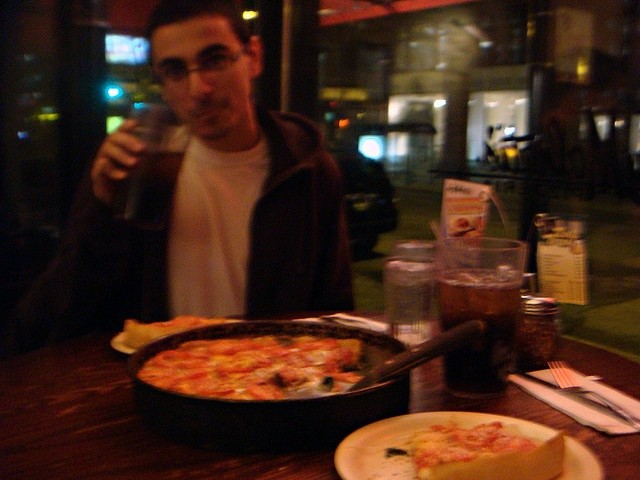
[547,359,640,431]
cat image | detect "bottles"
[521,298,562,368]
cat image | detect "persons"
[16,0,355,341]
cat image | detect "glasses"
[158,48,248,80]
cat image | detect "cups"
[438,238,527,406]
[384,255,438,348]
[390,238,434,260]
[123,102,197,230]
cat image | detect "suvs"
[328,139,398,263]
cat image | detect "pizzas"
[137,333,365,401]
[411,421,545,464]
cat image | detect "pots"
[128,321,410,447]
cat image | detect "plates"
[334,411,603,480]
[110,318,245,355]
